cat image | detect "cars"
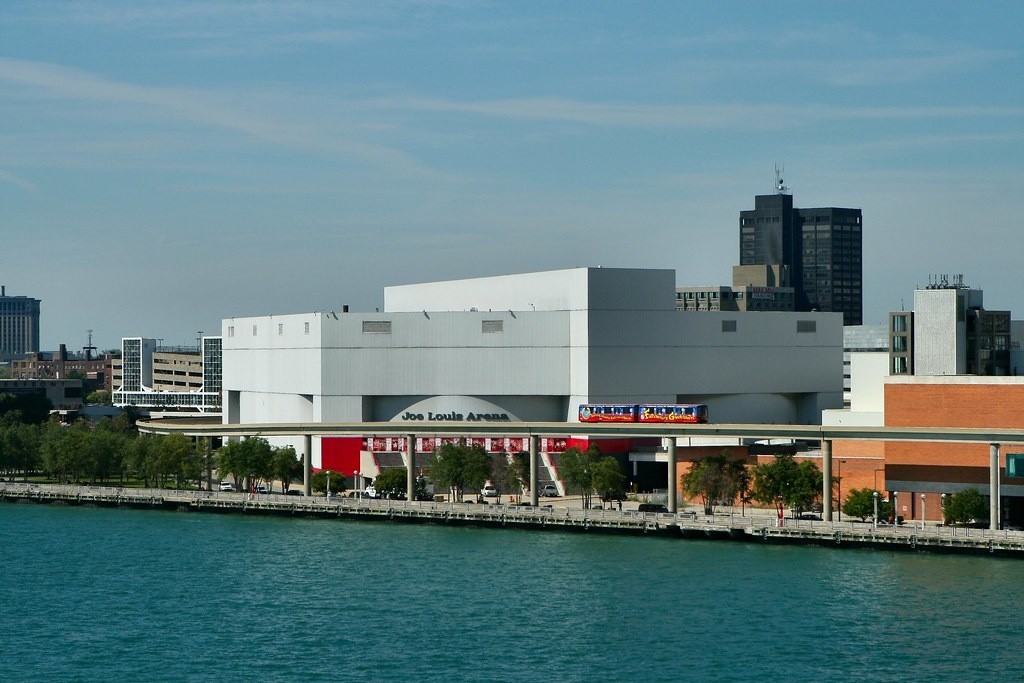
[480,485,496,497]
[639,504,668,513]
[543,485,558,497]
[217,482,232,491]
[284,490,304,496]
[936,524,951,527]
[254,486,268,493]
[802,514,823,521]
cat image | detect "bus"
[578,404,709,424]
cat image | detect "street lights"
[921,494,926,531]
[354,470,358,500]
[838,459,846,522]
[873,492,878,528]
[942,494,946,523]
[326,471,331,493]
[359,472,363,501]
[893,492,899,526]
